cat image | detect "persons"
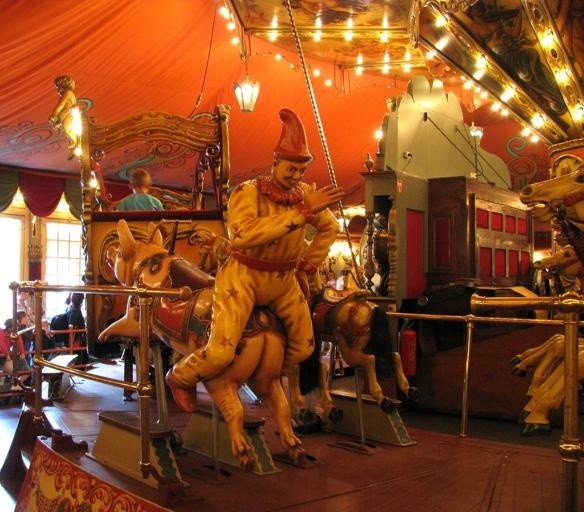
[115,166,164,210]
[165,108,344,412]
[0,291,35,396]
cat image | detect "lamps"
[233,24,261,113]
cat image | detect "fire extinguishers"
[399,319,416,376]
